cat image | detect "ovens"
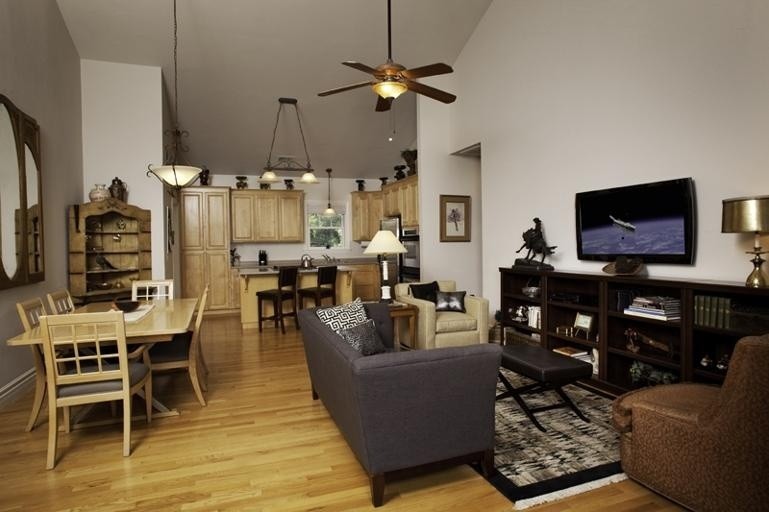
[399,227,419,285]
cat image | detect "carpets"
[467,368,629,511]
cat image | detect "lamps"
[372,82,408,99]
[721,196,768,288]
[256,97,320,185]
[364,230,409,300]
[146,0,207,190]
[324,168,336,217]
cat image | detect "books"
[624,296,682,321]
[552,346,588,357]
[694,295,730,329]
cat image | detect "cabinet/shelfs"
[399,181,418,227]
[688,283,769,388]
[278,190,304,243]
[351,192,383,240]
[67,198,152,307]
[231,189,278,244]
[499,268,544,348]
[382,185,399,217]
[178,186,232,250]
[230,269,241,309]
[604,276,687,397]
[544,271,604,385]
[180,251,231,311]
[27,204,41,274]
[14,209,24,270]
[351,271,380,300]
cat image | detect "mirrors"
[1,94,46,291]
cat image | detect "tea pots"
[301,254,314,267]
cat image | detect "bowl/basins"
[116,301,139,312]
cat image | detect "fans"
[316,0,457,111]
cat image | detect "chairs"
[132,278,174,301]
[255,266,300,334]
[47,288,74,314]
[142,282,206,423]
[14,297,71,431]
[297,266,338,310]
[39,311,152,469]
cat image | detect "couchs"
[394,279,490,351]
[610,334,769,511]
[298,301,503,507]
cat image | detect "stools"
[495,339,593,432]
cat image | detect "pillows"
[435,290,466,313]
[409,280,440,304]
[316,297,368,332]
[336,317,386,357]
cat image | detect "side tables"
[374,301,418,349]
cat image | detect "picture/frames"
[439,195,472,242]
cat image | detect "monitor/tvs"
[576,176,697,273]
